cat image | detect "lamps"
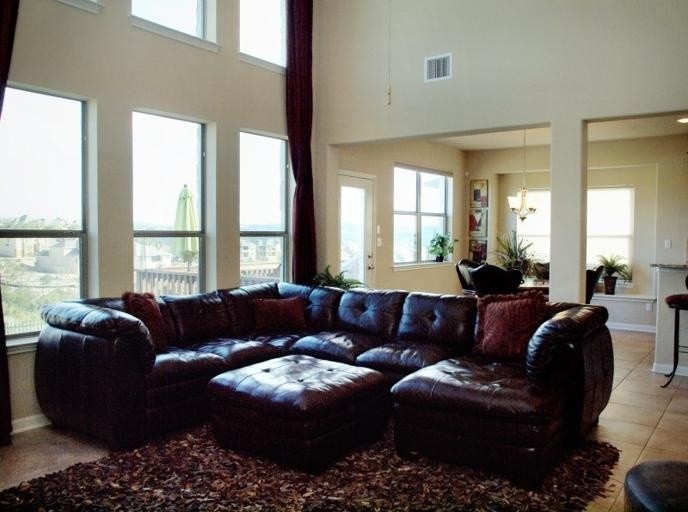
[533,261,550,280]
[586,266,605,303]
[455,258,523,298]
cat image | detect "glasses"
[511,128,536,221]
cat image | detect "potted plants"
[467,177,489,263]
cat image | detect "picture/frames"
[518,279,549,295]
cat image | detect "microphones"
[657,291,688,385]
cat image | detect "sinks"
[32,278,613,492]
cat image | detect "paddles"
[2,423,621,511]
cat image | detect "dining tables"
[596,253,633,295]
[428,233,459,261]
